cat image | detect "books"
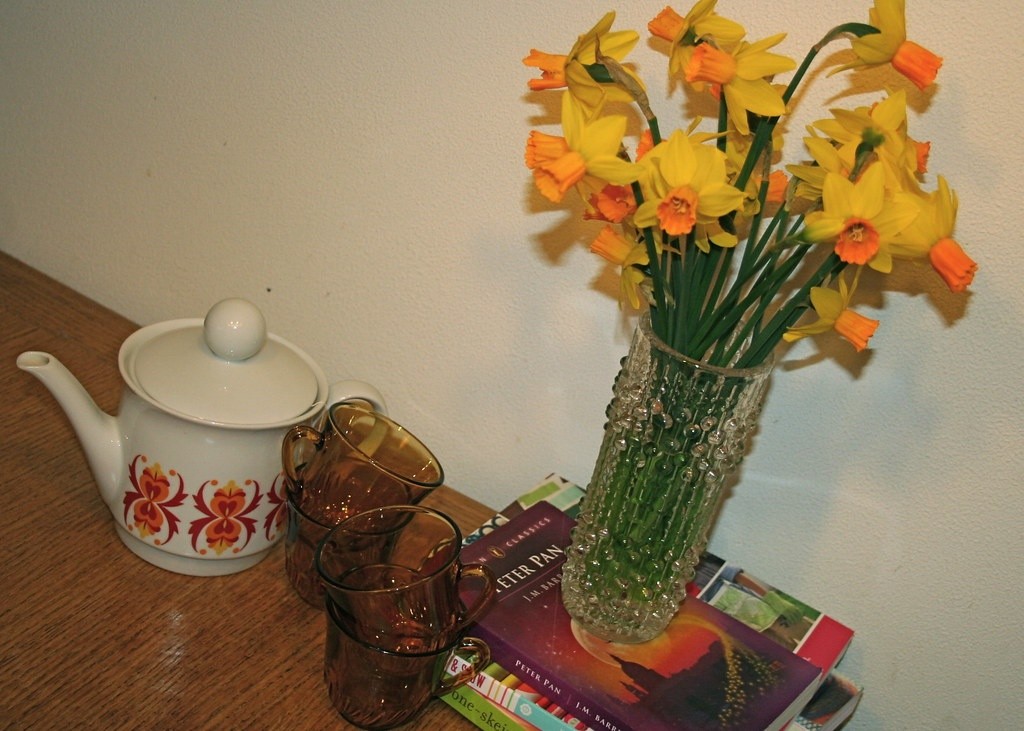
[457,472,854,730]
[443,654,864,731]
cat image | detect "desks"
[0,249,498,731]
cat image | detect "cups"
[315,504,498,654]
[325,563,490,731]
[282,401,444,544]
[285,456,416,613]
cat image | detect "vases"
[562,311,775,644]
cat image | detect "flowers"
[523,0,978,599]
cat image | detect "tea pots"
[18,297,388,577]
